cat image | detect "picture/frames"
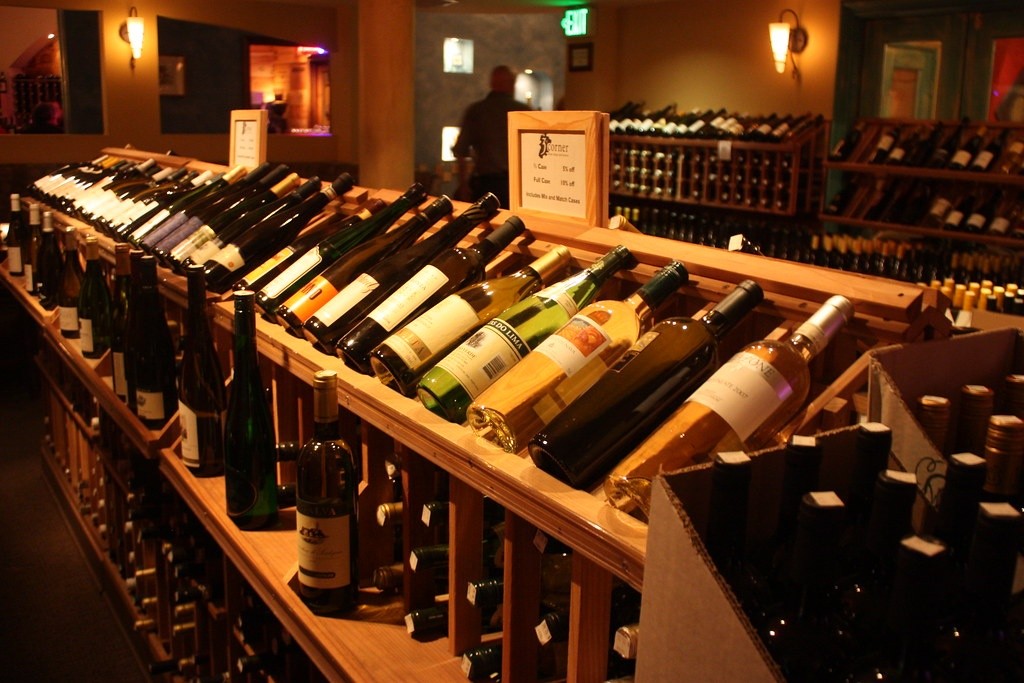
[567,41,594,72]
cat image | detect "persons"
[452,65,534,210]
[16,104,64,134]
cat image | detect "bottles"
[10,155,523,478]
[466,261,688,453]
[278,482,296,509]
[607,101,1024,318]
[296,371,357,615]
[123,491,268,683]
[528,280,763,490]
[415,245,634,423]
[715,374,1024,683]
[368,245,570,396]
[371,491,638,683]
[606,294,854,525]
[224,290,275,530]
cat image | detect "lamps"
[119,6,144,59]
[768,8,807,73]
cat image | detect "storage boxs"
[634,420,1024,683]
[866,326,1024,532]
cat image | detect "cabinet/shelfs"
[1,115,1024,683]
